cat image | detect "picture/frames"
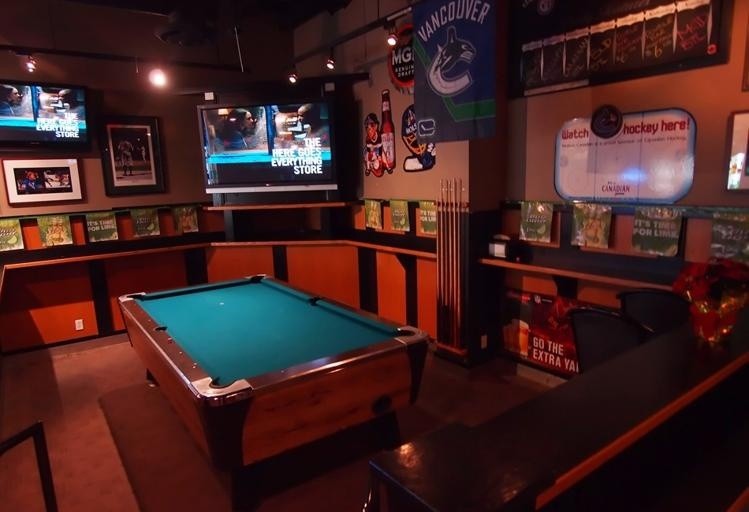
[96,114,167,197]
[2,158,84,205]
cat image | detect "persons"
[53,88,83,125]
[20,172,39,189]
[1,84,25,116]
[362,113,396,176]
[211,108,261,151]
[294,103,331,148]
[118,138,134,176]
[140,145,147,161]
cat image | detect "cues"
[436,179,461,349]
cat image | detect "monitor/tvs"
[0,79,90,153]
[196,97,340,195]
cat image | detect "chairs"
[567,289,691,374]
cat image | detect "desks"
[118,273,433,511]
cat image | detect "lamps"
[25,53,37,73]
[325,47,336,71]
[288,68,298,84]
[387,20,399,47]
